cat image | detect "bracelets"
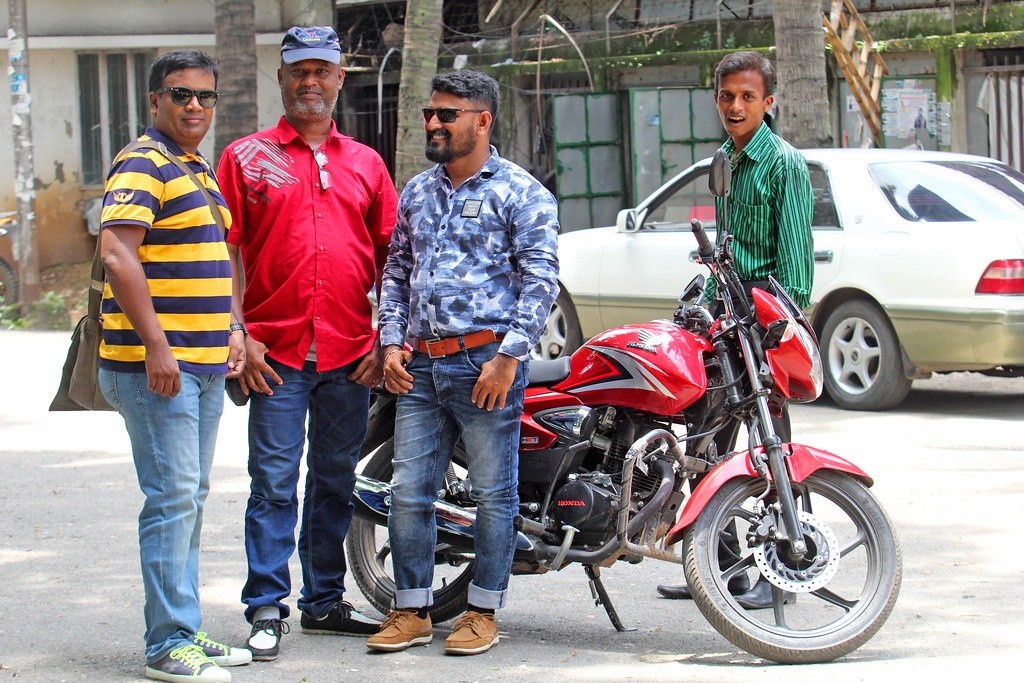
[229,322,247,337]
[384,349,401,361]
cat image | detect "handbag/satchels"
[48,313,116,411]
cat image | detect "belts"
[413,327,505,358]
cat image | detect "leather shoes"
[657,571,750,599]
[733,579,797,608]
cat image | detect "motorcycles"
[343,218,904,665]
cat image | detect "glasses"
[422,107,492,123]
[156,86,220,108]
[313,149,332,190]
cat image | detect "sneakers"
[191,631,252,668]
[244,618,290,661]
[300,601,384,637]
[145,644,232,683]
[444,612,499,654]
[366,609,433,651]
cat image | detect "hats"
[280,26,341,65]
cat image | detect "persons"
[366,70,560,655]
[98,49,252,683]
[215,26,399,661]
[657,50,814,609]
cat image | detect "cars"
[527,148,1024,410]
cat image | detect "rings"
[372,380,376,383]
